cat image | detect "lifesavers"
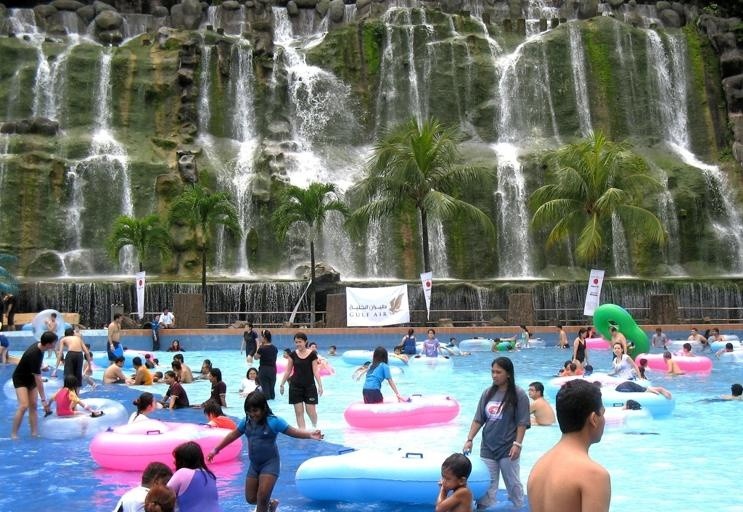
[547,373,674,427]
[90,353,147,369]
[276,363,289,373]
[317,368,335,376]
[639,334,743,375]
[342,350,408,380]
[295,448,492,504]
[344,394,459,428]
[459,337,546,351]
[88,420,242,472]
[23,308,71,343]
[36,397,128,441]
[586,304,650,361]
[3,375,60,400]
[408,342,459,373]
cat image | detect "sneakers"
[269,498,279,512]
